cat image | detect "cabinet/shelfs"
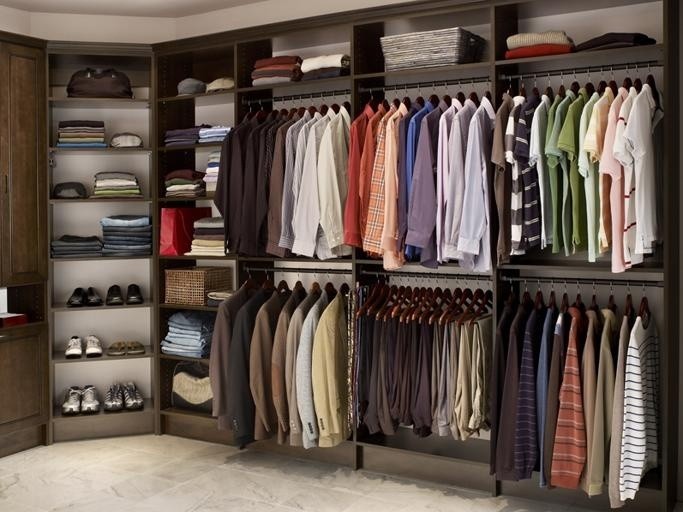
[0,43,47,286]
[0,322,49,459]
[356,1,498,495]
[500,0,683,512]
[157,33,236,446]
[234,10,353,468]
[48,44,155,444]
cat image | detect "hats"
[177,78,205,97]
[110,134,143,147]
[55,182,86,198]
[205,78,235,94]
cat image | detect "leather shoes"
[86,286,104,308]
[67,286,82,307]
[126,284,144,305]
[106,285,123,304]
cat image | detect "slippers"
[127,341,145,354]
[107,341,125,355]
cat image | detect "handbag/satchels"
[66,68,133,99]
[171,362,213,413]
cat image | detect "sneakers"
[104,383,123,410]
[81,385,100,413]
[61,385,80,414]
[86,335,103,356]
[123,383,144,410]
[64,336,82,359]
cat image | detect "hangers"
[507,276,651,318]
[240,263,351,300]
[356,272,493,325]
[240,89,350,121]
[366,76,492,109]
[501,70,656,95]
